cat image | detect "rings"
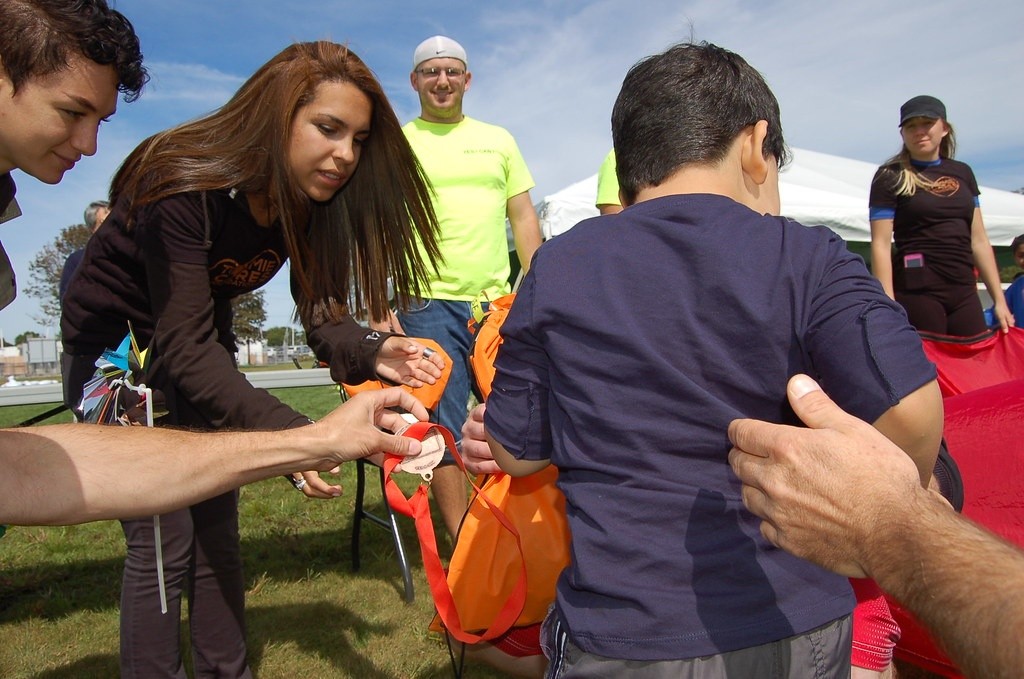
[423,347,437,360]
[294,477,306,491]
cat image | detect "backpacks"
[342,296,576,647]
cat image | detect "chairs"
[341,374,422,601]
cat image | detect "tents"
[506,146,1023,252]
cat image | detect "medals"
[395,422,444,481]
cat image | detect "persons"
[0,0,152,310]
[461,42,944,679]
[869,94,1024,333]
[59,40,445,679]
[364,36,544,551]
[0,387,429,527]
[727,374,1024,679]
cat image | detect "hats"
[413,36,469,71]
[897,96,947,126]
